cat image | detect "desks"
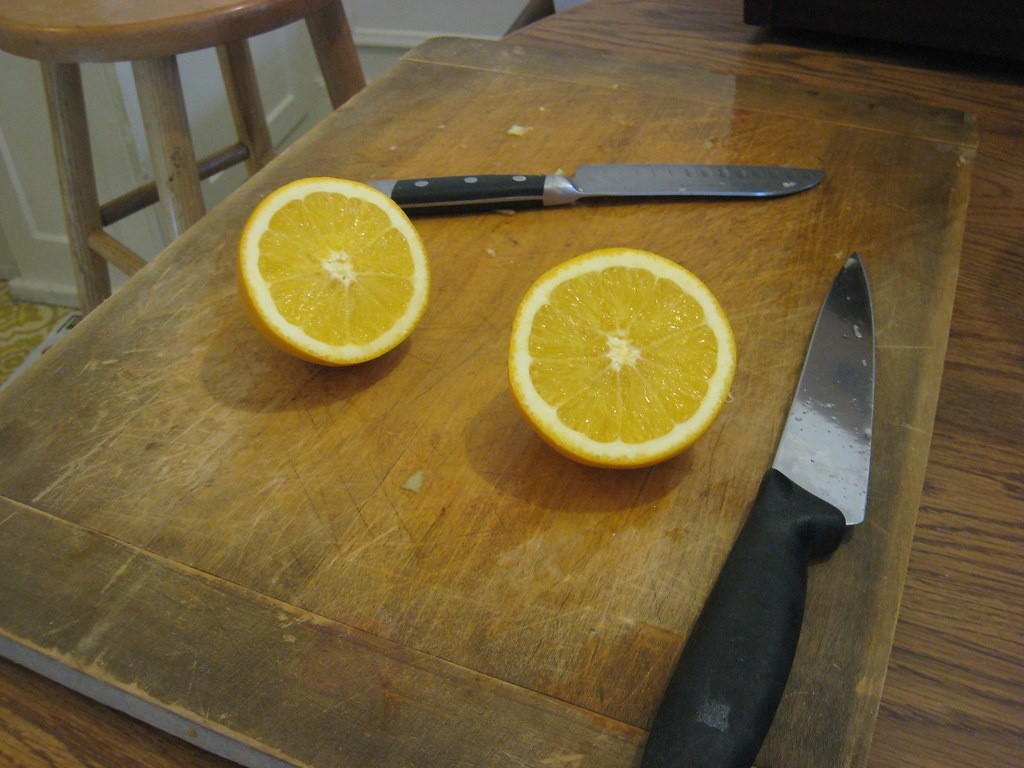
[0,4,1023,768]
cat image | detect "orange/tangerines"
[237,177,431,366]
[507,246,737,470]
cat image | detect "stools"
[0,2,369,325]
